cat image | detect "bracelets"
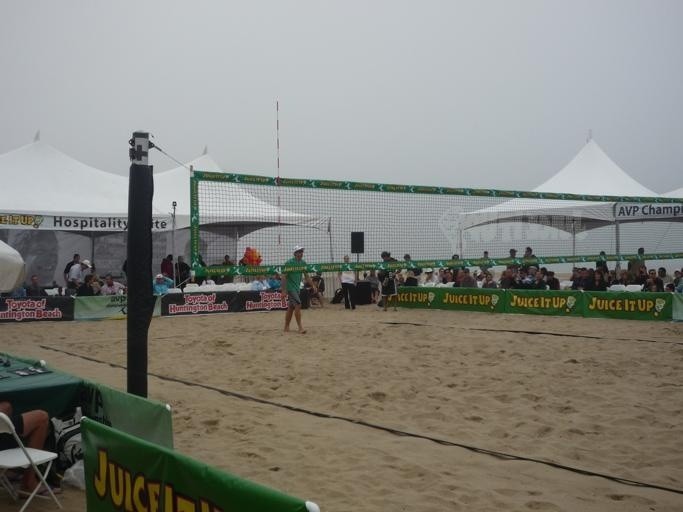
[354,280,356,283]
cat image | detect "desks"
[0,355,84,487]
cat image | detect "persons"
[280,246,317,336]
[364,270,380,304]
[9,246,281,296]
[11,230,58,286]
[0,400,62,499]
[376,250,402,312]
[303,271,327,309]
[337,256,358,311]
[396,246,682,294]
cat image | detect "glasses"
[649,272,656,274]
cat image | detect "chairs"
[0,411,63,511]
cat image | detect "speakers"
[351,232,364,253]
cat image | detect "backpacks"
[49,406,85,475]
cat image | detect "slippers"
[14,483,64,500]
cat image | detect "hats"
[80,259,92,269]
[673,268,681,274]
[291,245,305,256]
[154,273,164,279]
[423,267,434,274]
